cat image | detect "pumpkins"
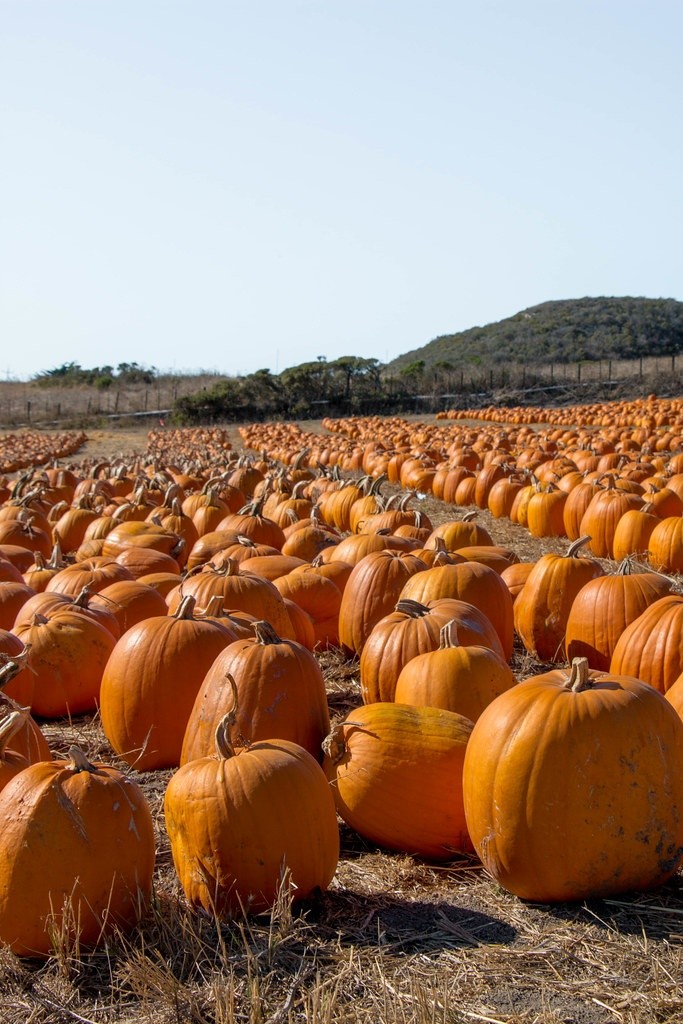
[1,385,682,965]
